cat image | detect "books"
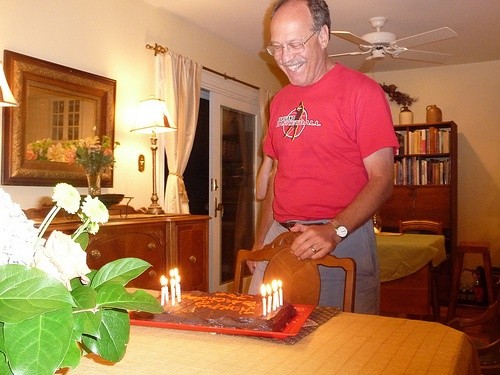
[392,127,452,186]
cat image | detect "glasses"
[264,32,316,57]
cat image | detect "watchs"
[327,220,349,242]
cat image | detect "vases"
[85,173,103,196]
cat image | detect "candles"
[159,268,181,306]
[260,279,284,317]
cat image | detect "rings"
[310,246,317,254]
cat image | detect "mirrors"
[0,49,116,188]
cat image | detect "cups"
[373,211,382,233]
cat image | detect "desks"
[71,288,478,375]
[372,232,444,317]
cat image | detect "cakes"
[131,290,296,332]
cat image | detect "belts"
[275,221,324,233]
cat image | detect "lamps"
[129,98,178,214]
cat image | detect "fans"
[327,17,458,74]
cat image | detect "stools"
[447,241,494,321]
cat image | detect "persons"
[247,0,399,316]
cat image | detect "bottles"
[426,105,442,122]
[399,106,413,124]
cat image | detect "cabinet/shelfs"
[28,215,213,293]
[377,121,458,303]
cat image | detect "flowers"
[66,137,120,178]
[27,139,67,163]
[0,182,164,375]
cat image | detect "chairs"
[233,232,356,312]
